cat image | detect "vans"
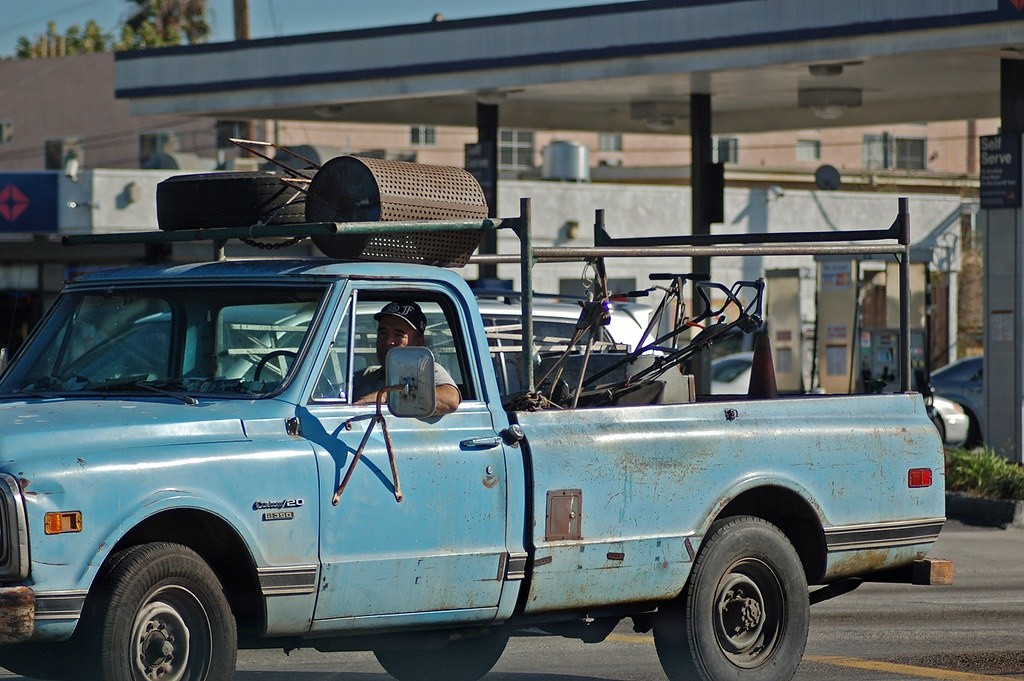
[27,300,661,417]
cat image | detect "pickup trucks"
[0,192,957,681]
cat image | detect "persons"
[352,300,462,415]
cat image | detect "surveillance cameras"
[65,159,78,177]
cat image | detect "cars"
[912,355,988,450]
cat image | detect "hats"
[374,302,426,332]
